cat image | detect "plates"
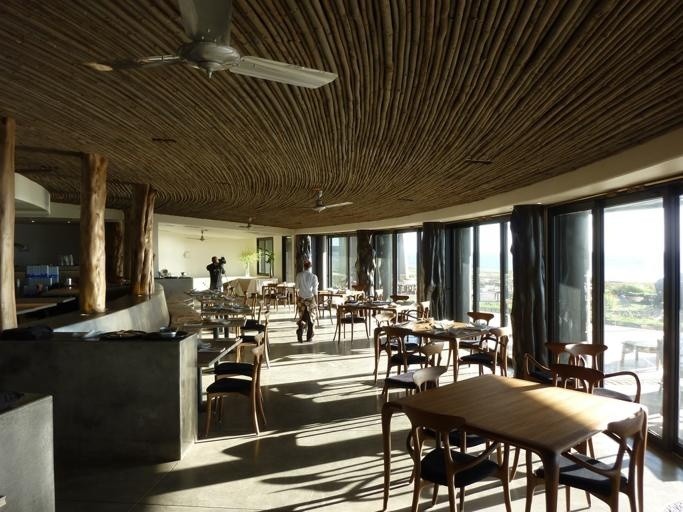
[196,339,226,353]
[412,316,493,335]
[324,285,350,295]
[182,288,236,301]
[202,303,247,313]
[183,317,232,325]
[344,294,416,306]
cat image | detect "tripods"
[216,265,229,291]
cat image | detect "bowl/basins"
[160,326,178,339]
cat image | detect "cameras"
[218,257,226,264]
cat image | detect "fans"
[83,1,339,95]
[182,229,211,242]
[234,217,264,231]
[293,186,354,214]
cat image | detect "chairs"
[263,282,364,326]
[183,275,270,437]
[333,296,508,395]
[381,341,649,512]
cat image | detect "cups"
[64,277,73,286]
[283,282,286,286]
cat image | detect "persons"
[206,257,225,291]
[294,260,319,343]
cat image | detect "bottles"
[55,252,74,267]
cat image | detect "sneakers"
[296,329,303,343]
[307,338,313,342]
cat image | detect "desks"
[618,340,660,370]
